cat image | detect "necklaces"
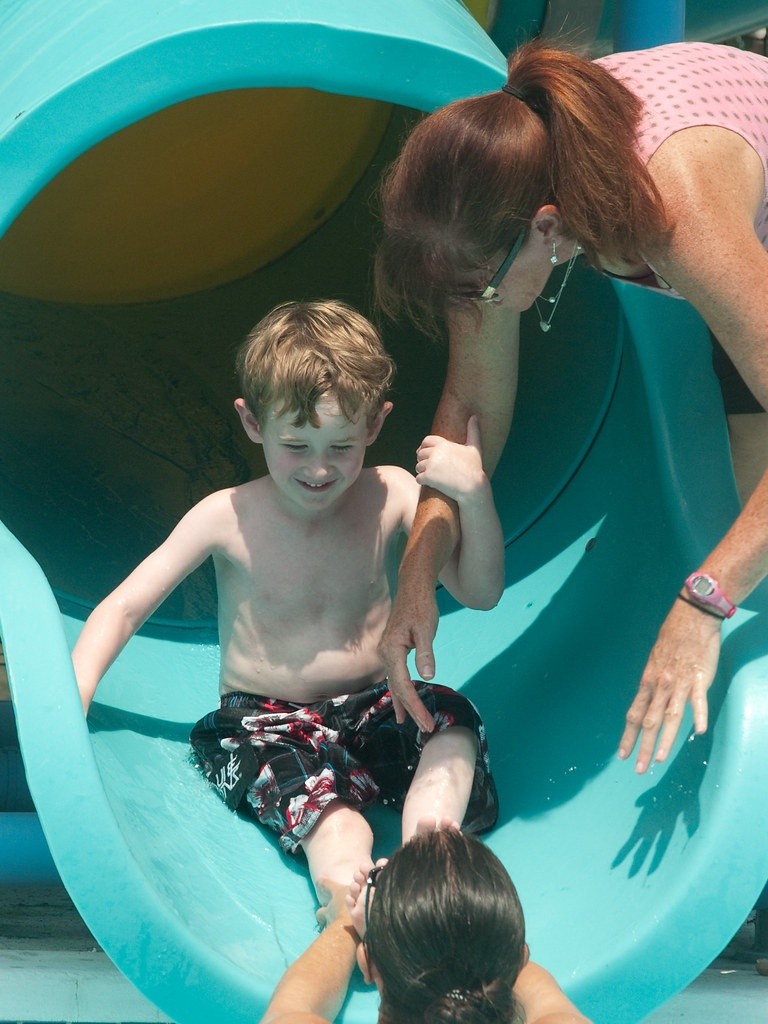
[525,231,583,332]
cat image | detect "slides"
[0,0,768,1024]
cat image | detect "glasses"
[450,224,527,301]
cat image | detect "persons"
[70,296,500,943]
[376,38,768,775]
[257,829,596,1024]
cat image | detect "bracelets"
[677,593,726,622]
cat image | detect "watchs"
[684,571,737,619]
